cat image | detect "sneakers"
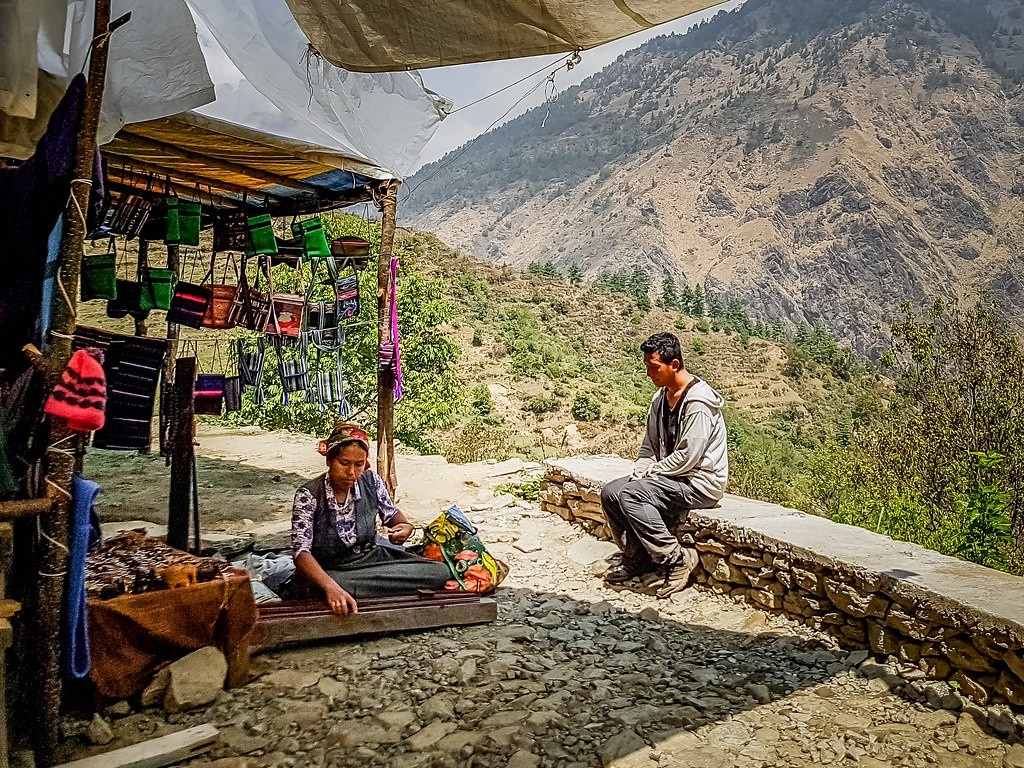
[655,546,699,598]
[603,548,653,583]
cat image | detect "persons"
[289,425,449,616]
[601,332,731,599]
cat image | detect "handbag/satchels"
[417,504,509,596]
[79,164,369,417]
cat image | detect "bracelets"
[404,522,416,538]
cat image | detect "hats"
[43,346,108,432]
